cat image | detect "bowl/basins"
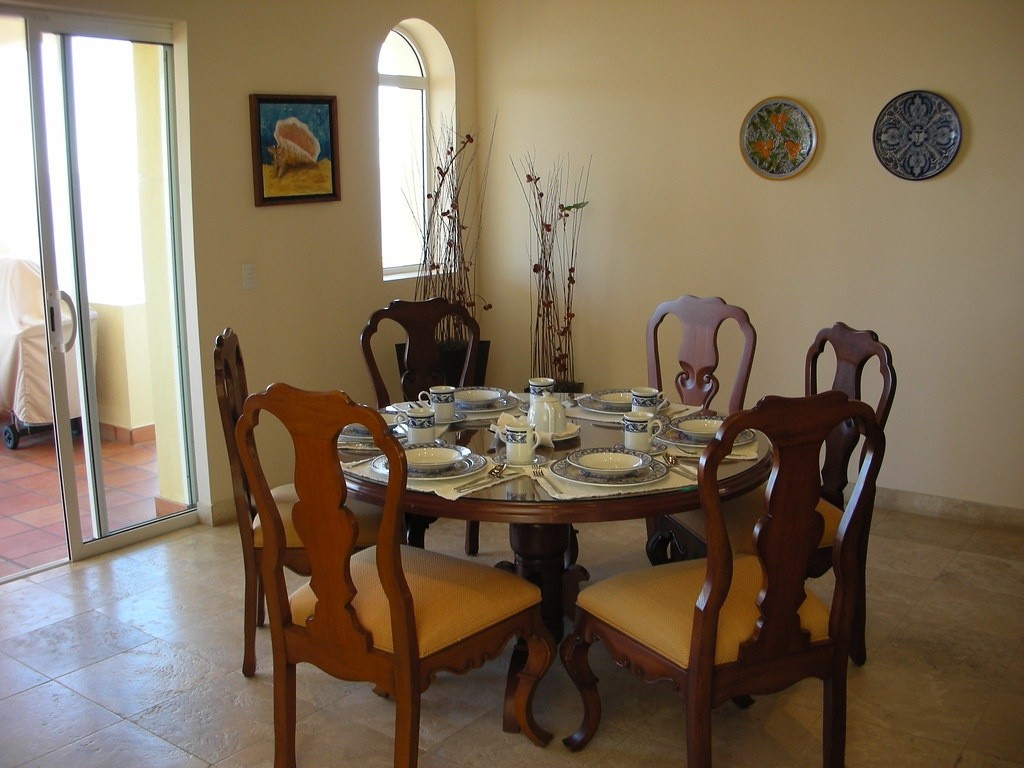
[393,442,472,472]
[451,386,507,407]
[668,415,734,439]
[566,447,653,479]
[345,412,400,435]
[589,389,634,410]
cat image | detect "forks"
[532,464,563,494]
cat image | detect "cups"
[630,386,668,419]
[529,377,556,411]
[623,411,663,454]
[394,407,436,444]
[418,386,456,420]
[504,425,541,465]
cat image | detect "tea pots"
[526,393,568,434]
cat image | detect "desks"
[343,392,773,734]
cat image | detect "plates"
[577,395,671,414]
[519,400,566,414]
[369,454,486,480]
[436,411,467,426]
[338,427,406,441]
[653,423,756,448]
[495,421,582,443]
[492,452,548,468]
[455,396,519,413]
[549,456,669,487]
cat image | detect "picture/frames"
[248,93,341,207]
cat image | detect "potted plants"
[509,145,590,394]
[395,107,491,403]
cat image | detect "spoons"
[453,463,509,493]
[662,453,698,478]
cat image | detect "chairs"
[213,326,409,677]
[361,297,480,554]
[560,294,898,768]
[234,382,557,768]
